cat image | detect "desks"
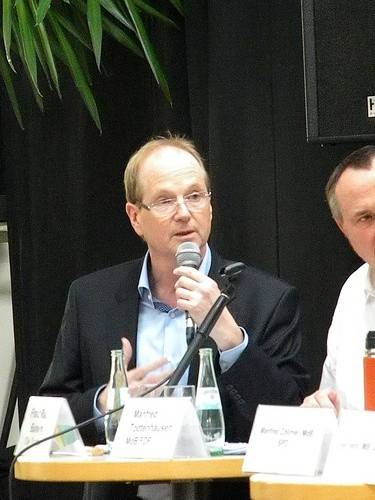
[14,446,255,500]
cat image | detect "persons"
[36,135,313,500]
[299,143,375,429]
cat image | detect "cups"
[137,385,162,398]
[163,385,195,398]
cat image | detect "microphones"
[175,242,203,345]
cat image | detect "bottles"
[193,348,226,454]
[364,330,375,410]
[104,350,131,449]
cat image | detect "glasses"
[135,189,212,217]
